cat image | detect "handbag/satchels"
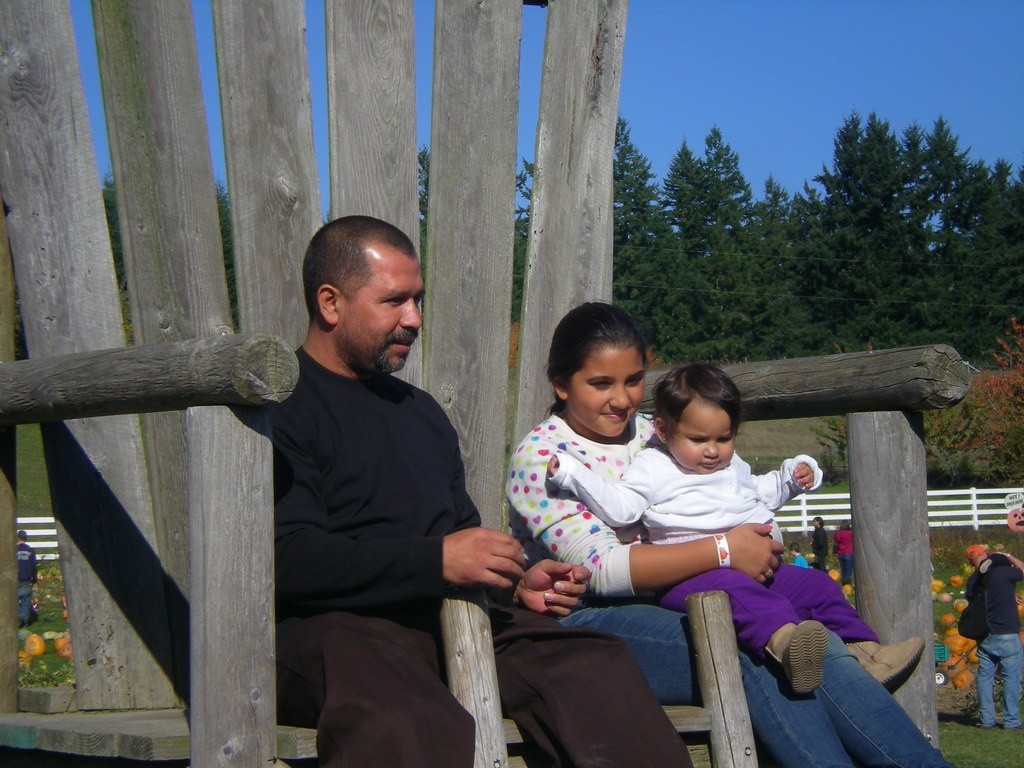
[958,587,989,641]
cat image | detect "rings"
[764,570,772,577]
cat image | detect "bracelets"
[1006,554,1009,557]
[713,534,730,568]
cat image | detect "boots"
[765,620,828,694]
[846,635,925,691]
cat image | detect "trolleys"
[933,644,977,686]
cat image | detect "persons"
[17,529,38,627]
[505,301,956,768]
[834,520,852,585]
[274,214,694,768]
[810,517,828,572]
[789,543,809,568]
[547,363,925,694]
[964,544,1024,729]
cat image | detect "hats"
[18,531,27,539]
[965,544,985,561]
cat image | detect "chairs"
[0,0,972,768]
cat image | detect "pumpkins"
[930,574,1024,689]
[827,568,840,581]
[842,584,853,595]
[17,634,72,667]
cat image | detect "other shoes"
[1006,724,1024,732]
[980,558,992,573]
[981,722,1003,730]
